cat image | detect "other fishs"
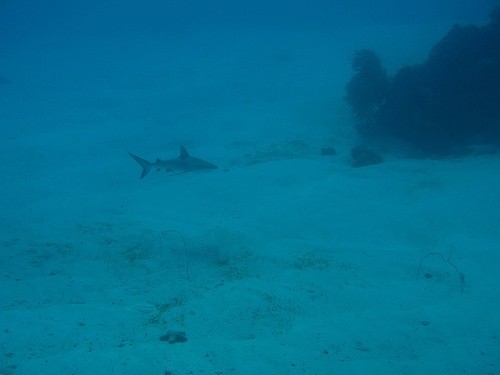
[126,147,218,179]
